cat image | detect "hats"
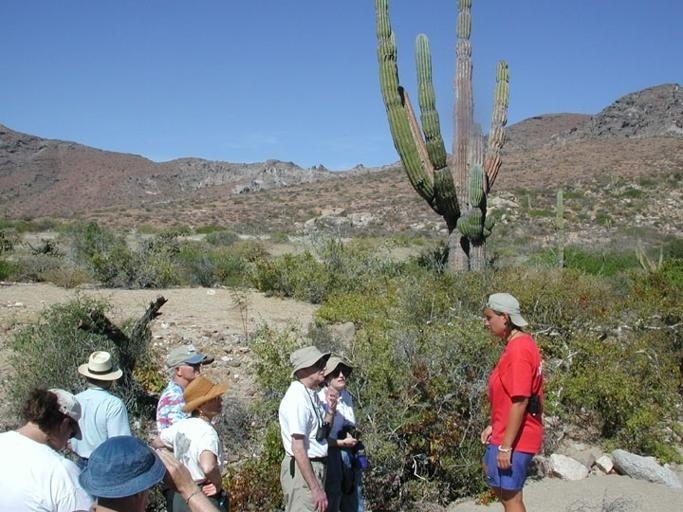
[485,292,528,327]
[76,351,123,381]
[77,434,167,499]
[181,376,228,412]
[43,388,82,441]
[322,355,353,378]
[289,345,331,379]
[166,347,208,368]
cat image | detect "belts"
[289,455,328,464]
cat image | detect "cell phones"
[218,495,229,509]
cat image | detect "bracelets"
[497,444,513,454]
[184,489,204,505]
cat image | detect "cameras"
[527,394,540,417]
[337,425,362,440]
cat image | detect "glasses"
[331,367,348,377]
[187,363,200,366]
[67,422,76,438]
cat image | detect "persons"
[481,293,544,511]
[0,390,93,512]
[155,379,229,512]
[65,436,219,512]
[71,352,131,466]
[316,358,362,509]
[156,346,211,434]
[277,345,329,511]
